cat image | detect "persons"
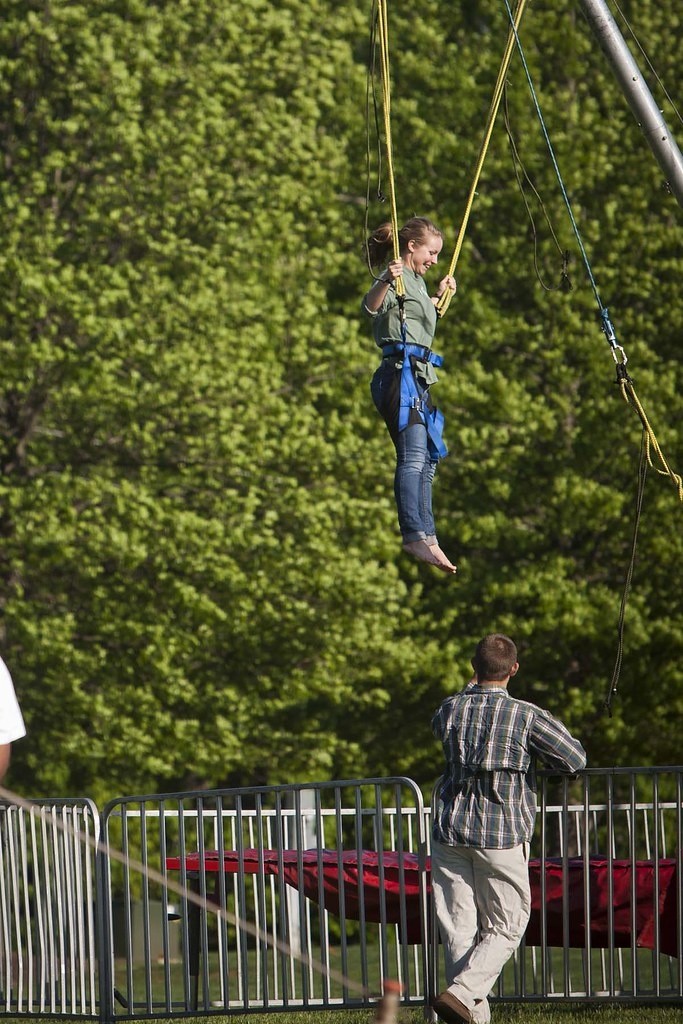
[0,657,27,786]
[361,217,458,574]
[431,634,586,1024]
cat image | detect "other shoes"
[433,992,477,1024]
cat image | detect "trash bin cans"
[163,904,184,959]
